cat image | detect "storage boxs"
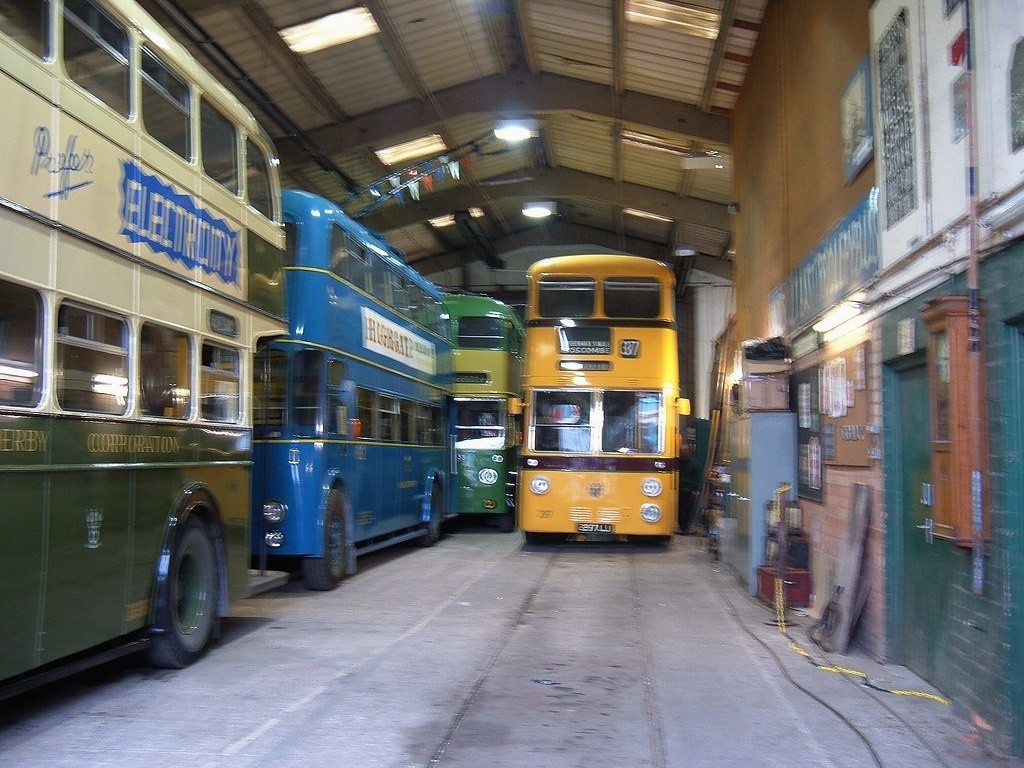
[736,374,788,414]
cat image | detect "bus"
[254,184,460,591]
[507,253,693,547]
[0,1,292,709]
[442,289,526,533]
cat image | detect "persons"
[678,443,705,534]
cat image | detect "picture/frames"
[838,51,873,188]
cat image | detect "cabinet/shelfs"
[728,413,799,596]
